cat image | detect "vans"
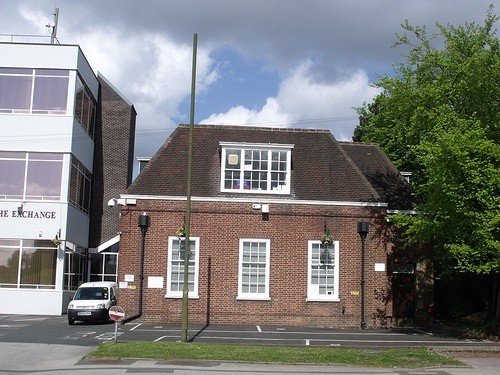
[67,281,117,324]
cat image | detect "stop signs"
[109,305,125,321]
